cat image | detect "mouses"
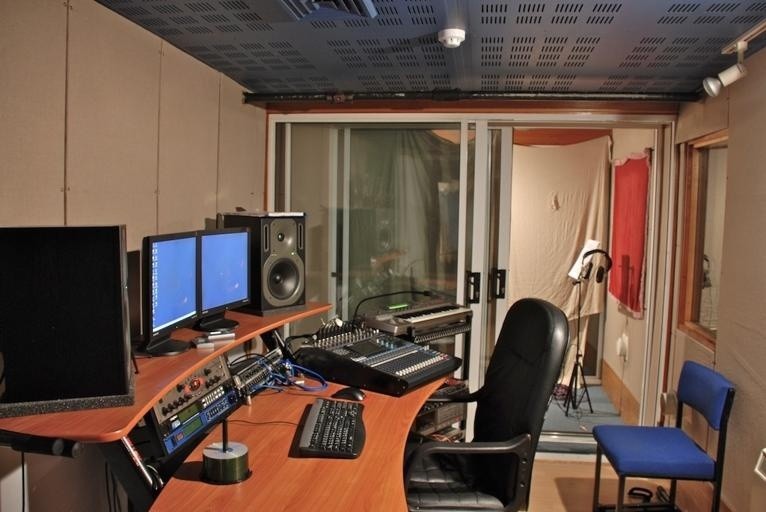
[335,388,366,401]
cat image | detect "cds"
[202,441,249,482]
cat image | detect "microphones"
[351,290,432,334]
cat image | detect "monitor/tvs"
[137,231,199,357]
[192,227,251,333]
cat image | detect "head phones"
[581,249,612,283]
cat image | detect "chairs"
[592,360,736,511]
[402,297,571,512]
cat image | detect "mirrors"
[678,129,729,353]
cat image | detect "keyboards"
[297,397,366,458]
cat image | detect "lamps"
[703,38,749,98]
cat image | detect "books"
[223,210,305,217]
[203,328,235,340]
[190,336,214,349]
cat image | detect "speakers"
[0,224,135,417]
[222,211,306,317]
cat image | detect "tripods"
[562,280,595,416]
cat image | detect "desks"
[0,301,451,512]
